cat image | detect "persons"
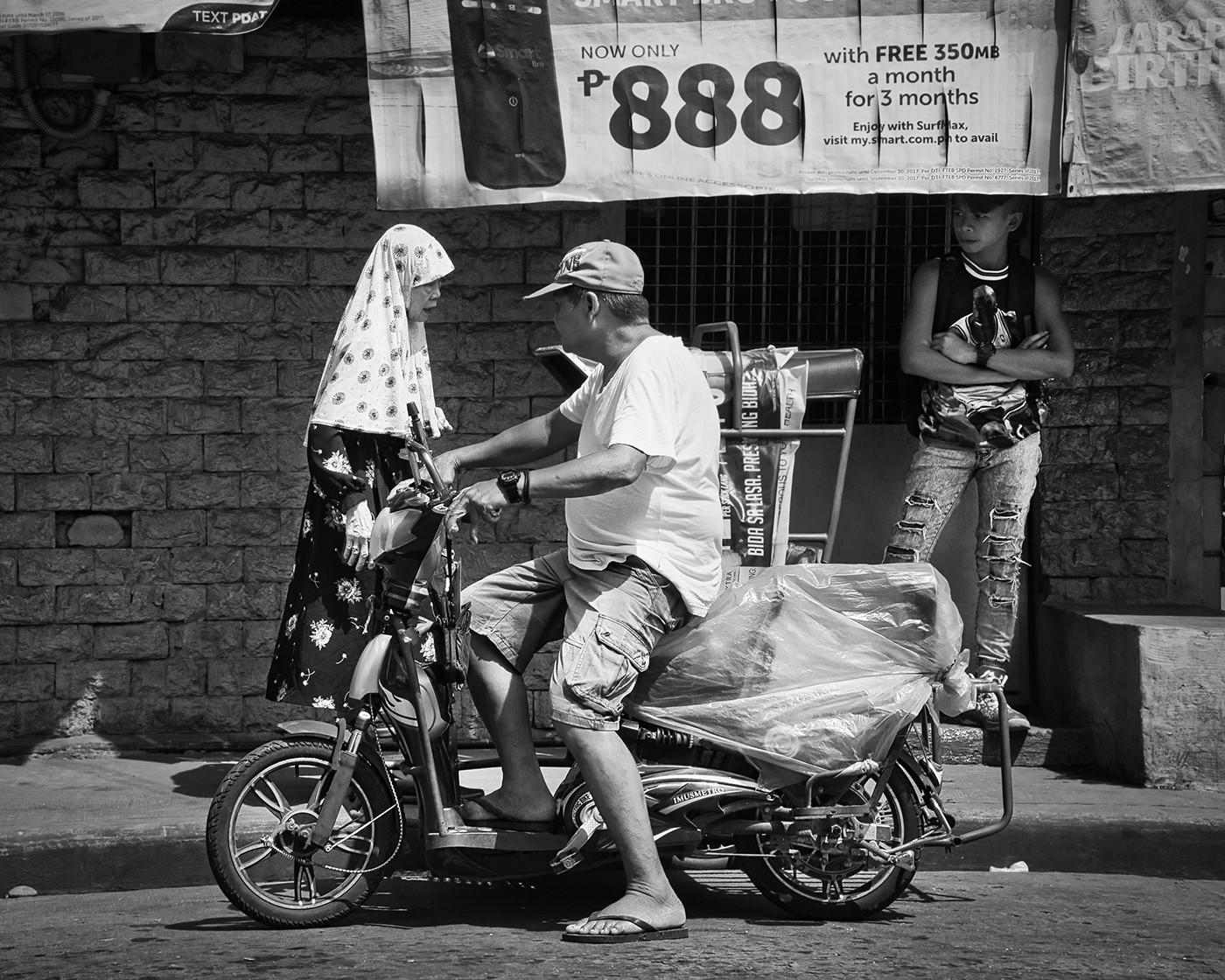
[387,238,726,943]
[882,194,1075,730]
[265,224,485,803]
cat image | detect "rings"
[353,546,359,554]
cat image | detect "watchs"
[498,468,522,504]
[974,341,997,367]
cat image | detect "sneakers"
[958,692,1031,732]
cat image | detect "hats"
[522,240,645,301]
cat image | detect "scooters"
[206,401,1014,928]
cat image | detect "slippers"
[562,910,690,944]
[452,797,560,834]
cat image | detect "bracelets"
[522,468,532,505]
